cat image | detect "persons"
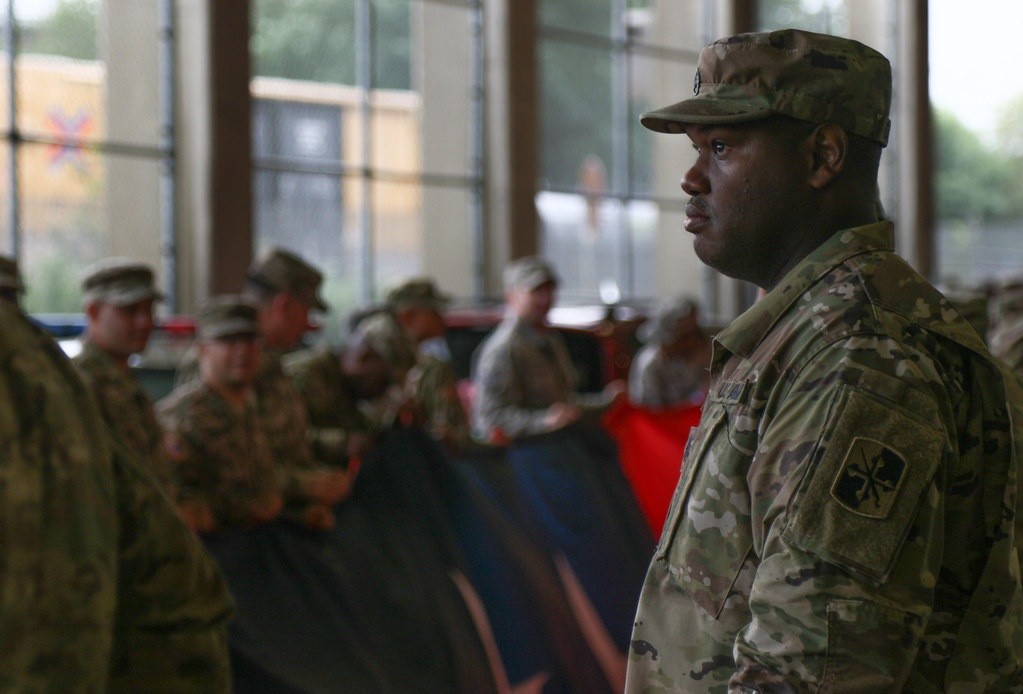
[932,275,1023,376]
[0,248,712,694]
[626,30,1023,694]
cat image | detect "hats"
[502,255,563,293]
[81,262,167,306]
[633,297,697,352]
[387,282,453,312]
[194,296,263,340]
[249,251,329,315]
[359,312,414,372]
[638,30,896,148]
